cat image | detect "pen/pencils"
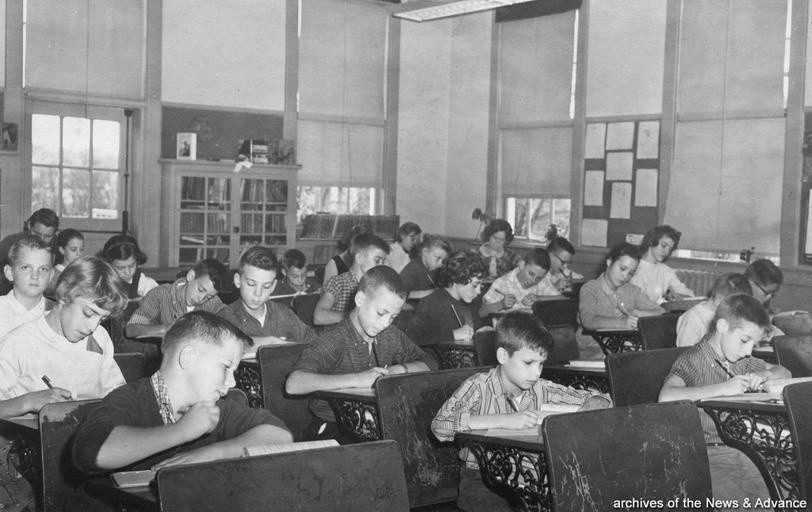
[42,375,56,390]
[619,303,631,317]
[494,288,506,297]
[715,358,734,379]
[177,405,192,412]
[557,267,567,279]
[506,396,518,413]
[450,304,463,329]
[426,273,439,289]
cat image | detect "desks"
[582,326,638,357]
[434,340,476,368]
[136,334,162,345]
[697,388,797,512]
[455,423,549,512]
[233,358,259,409]
[0,415,39,488]
[313,386,382,440]
[542,361,608,394]
[752,342,774,363]
[85,472,153,508]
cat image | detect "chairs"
[295,294,320,325]
[375,364,491,510]
[542,400,713,512]
[771,333,812,376]
[475,325,500,368]
[114,351,146,385]
[772,310,812,334]
[604,346,690,407]
[638,313,682,348]
[257,344,314,437]
[781,380,812,512]
[532,299,578,360]
[39,399,117,512]
[154,440,413,512]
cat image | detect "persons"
[0,208,812,512]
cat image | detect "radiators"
[674,268,718,297]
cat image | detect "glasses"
[555,254,573,266]
[753,280,776,297]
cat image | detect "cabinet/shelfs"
[296,236,394,266]
[156,156,302,269]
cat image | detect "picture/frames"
[0,119,21,155]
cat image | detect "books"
[179,176,287,267]
[299,212,400,240]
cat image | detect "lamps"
[390,0,512,24]
